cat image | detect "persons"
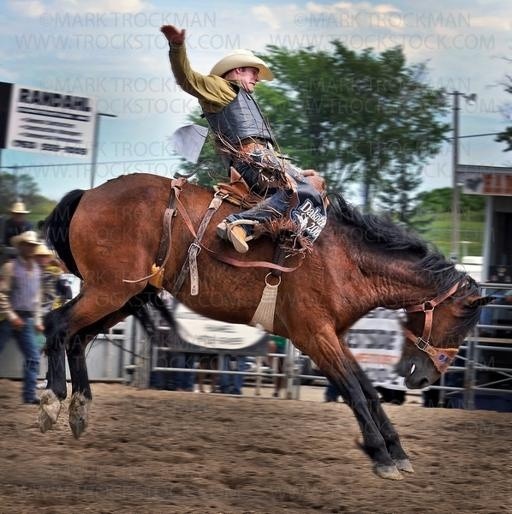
[158,23,316,254]
[0,203,287,405]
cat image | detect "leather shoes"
[216,216,252,254]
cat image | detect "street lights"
[431,81,481,264]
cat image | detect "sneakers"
[23,397,40,406]
[36,379,51,390]
[155,385,243,395]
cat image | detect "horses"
[32,171,500,483]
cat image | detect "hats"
[9,230,43,249]
[209,49,276,82]
[7,200,34,215]
[30,243,56,257]
